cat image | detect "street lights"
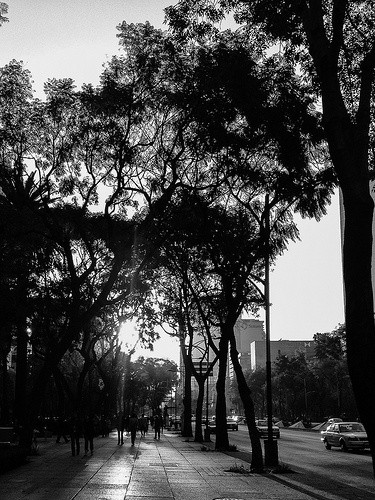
[179,336,223,442]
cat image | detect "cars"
[320,422,370,452]
[167,414,218,434]
[227,418,238,431]
[255,420,280,439]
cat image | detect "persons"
[146,414,153,426]
[158,414,164,433]
[69,414,83,457]
[99,414,131,438]
[152,415,161,440]
[138,413,149,436]
[127,414,139,446]
[115,412,126,445]
[81,414,96,456]
[56,417,70,444]
